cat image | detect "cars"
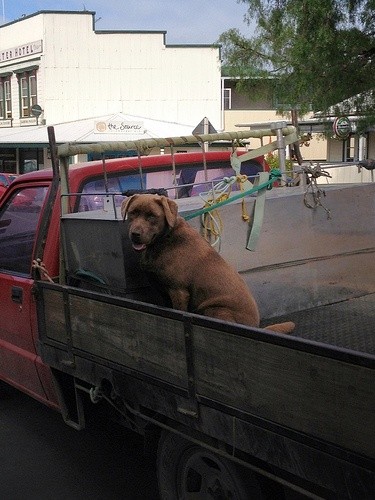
[0,173,42,212]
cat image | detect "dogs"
[121,194,294,334]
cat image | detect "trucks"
[0,120,375,500]
